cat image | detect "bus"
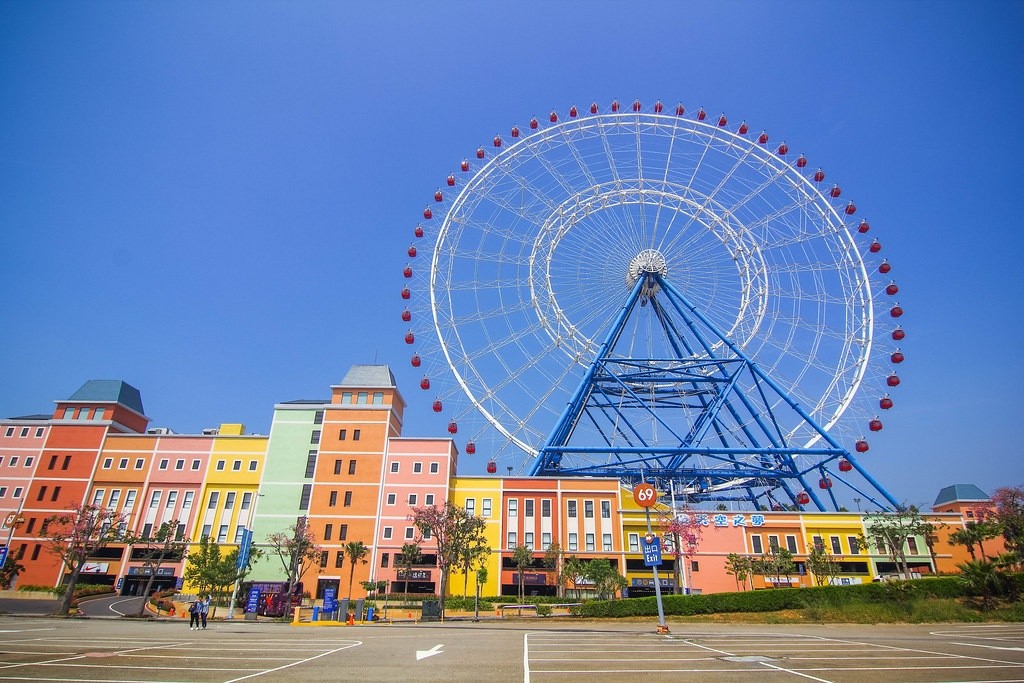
[238,580,304,618]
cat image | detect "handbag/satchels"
[188,602,197,612]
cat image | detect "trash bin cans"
[312,607,319,621]
[367,608,374,621]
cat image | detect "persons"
[201,595,213,630]
[190,595,203,630]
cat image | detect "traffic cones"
[348,614,355,626]
[408,611,412,619]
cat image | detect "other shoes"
[195,627,199,631]
[200,627,205,630]
[190,627,194,631]
